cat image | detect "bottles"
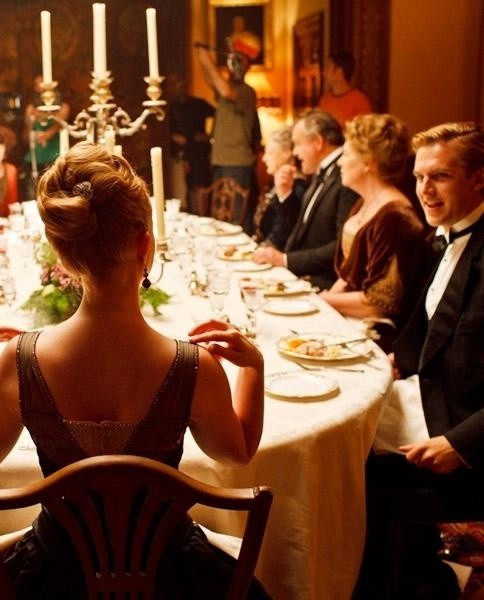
[0,197,42,311]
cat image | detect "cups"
[165,196,264,341]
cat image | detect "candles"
[93,3,106,74]
[150,146,165,238]
[146,8,159,78]
[59,130,69,157]
[41,10,52,86]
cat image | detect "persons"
[161,72,216,208]
[0,140,264,600]
[249,112,425,343]
[195,36,262,234]
[0,127,18,217]
[23,76,70,201]
[220,11,261,64]
[318,51,371,129]
[365,122,484,600]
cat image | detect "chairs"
[193,177,251,227]
[0,455,273,600]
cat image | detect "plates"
[275,331,373,361]
[236,260,273,271]
[262,301,318,316]
[264,283,312,296]
[262,369,339,399]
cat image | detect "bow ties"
[433,233,452,248]
[313,169,324,181]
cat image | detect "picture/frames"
[293,13,323,107]
[207,0,272,73]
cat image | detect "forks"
[294,362,365,373]
